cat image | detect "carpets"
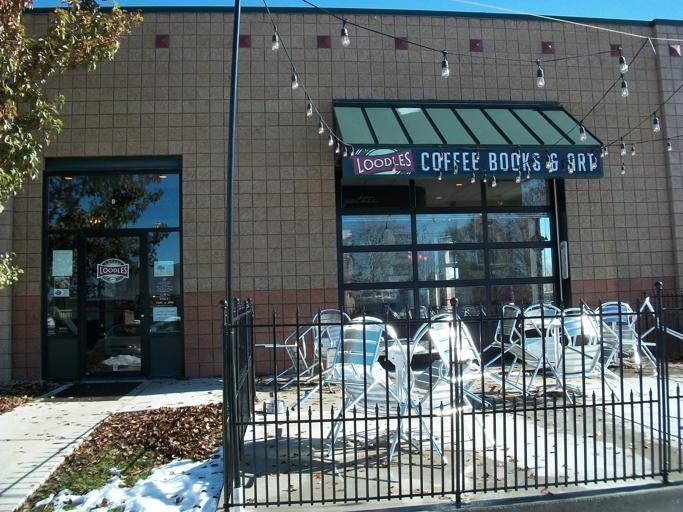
[40,381,142,402]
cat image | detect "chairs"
[266,321,334,409]
[541,307,618,404]
[627,302,670,372]
[592,302,639,370]
[324,314,416,463]
[312,310,350,381]
[522,302,565,331]
[480,304,539,382]
[409,311,495,462]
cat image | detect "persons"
[385,289,413,320]
